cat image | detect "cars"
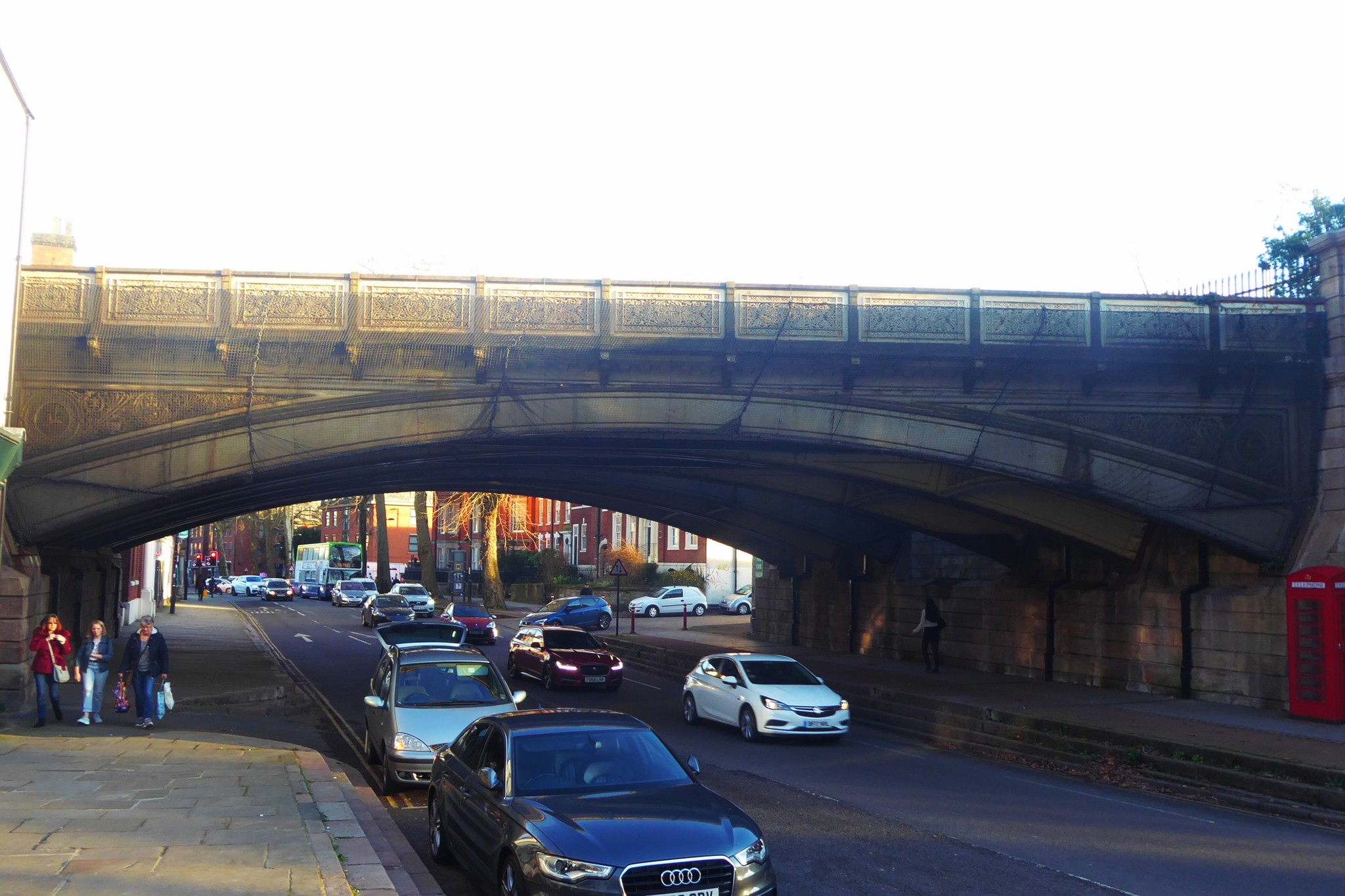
[361,593,417,629]
[426,708,780,896]
[231,575,267,597]
[363,618,528,797]
[440,601,498,646]
[349,578,379,598]
[259,580,295,602]
[331,579,368,607]
[719,584,753,615]
[682,652,851,744]
[204,576,240,595]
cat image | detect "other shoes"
[134,718,145,726]
[34,722,45,728]
[923,667,930,671]
[142,717,154,728]
[92,715,102,723]
[932,669,940,673]
[77,716,90,725]
[54,708,63,721]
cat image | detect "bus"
[294,541,365,601]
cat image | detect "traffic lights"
[190,551,218,568]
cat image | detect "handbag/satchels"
[155,678,166,720]
[53,664,69,683]
[155,682,174,709]
[114,677,130,713]
[937,618,945,631]
[125,670,133,685]
[203,589,209,598]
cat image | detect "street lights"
[390,508,399,528]
[290,533,302,577]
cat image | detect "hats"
[585,582,589,585]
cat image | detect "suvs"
[387,583,436,618]
[518,595,614,630]
[628,585,708,617]
[507,625,624,694]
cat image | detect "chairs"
[397,675,427,702]
[448,675,482,698]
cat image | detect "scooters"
[203,582,223,595]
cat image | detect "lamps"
[593,534,597,539]
[565,537,569,545]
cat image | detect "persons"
[582,730,646,783]
[580,582,592,595]
[74,620,114,725]
[30,614,72,728]
[736,667,757,683]
[195,574,207,601]
[375,575,405,586]
[118,615,169,729]
[208,576,216,598]
[909,598,940,673]
